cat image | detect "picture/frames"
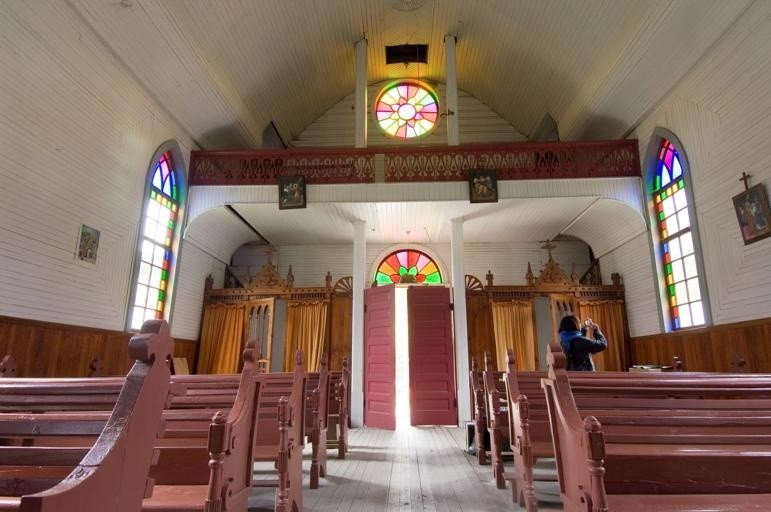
[733,184,771,245]
[278,175,307,208]
[72,223,102,270]
[469,169,498,203]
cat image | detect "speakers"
[385,43,428,65]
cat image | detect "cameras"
[583,318,592,326]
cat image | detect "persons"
[557,315,608,371]
